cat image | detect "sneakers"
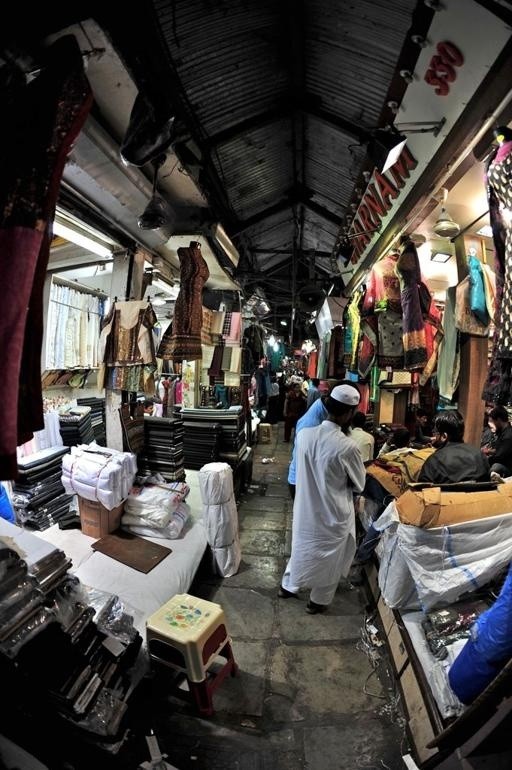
[277,585,296,599]
[305,599,327,615]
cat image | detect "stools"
[144,591,240,685]
[147,625,242,718]
[256,423,271,442]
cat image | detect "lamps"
[136,152,171,232]
[431,186,461,240]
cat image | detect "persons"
[143,400,157,417]
[267,369,321,443]
[277,384,366,614]
[346,412,376,468]
[155,375,182,417]
[414,409,437,443]
[376,429,418,458]
[484,406,512,478]
[288,379,362,500]
[347,234,431,377]
[157,241,210,360]
[416,410,491,483]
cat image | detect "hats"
[330,384,360,406]
